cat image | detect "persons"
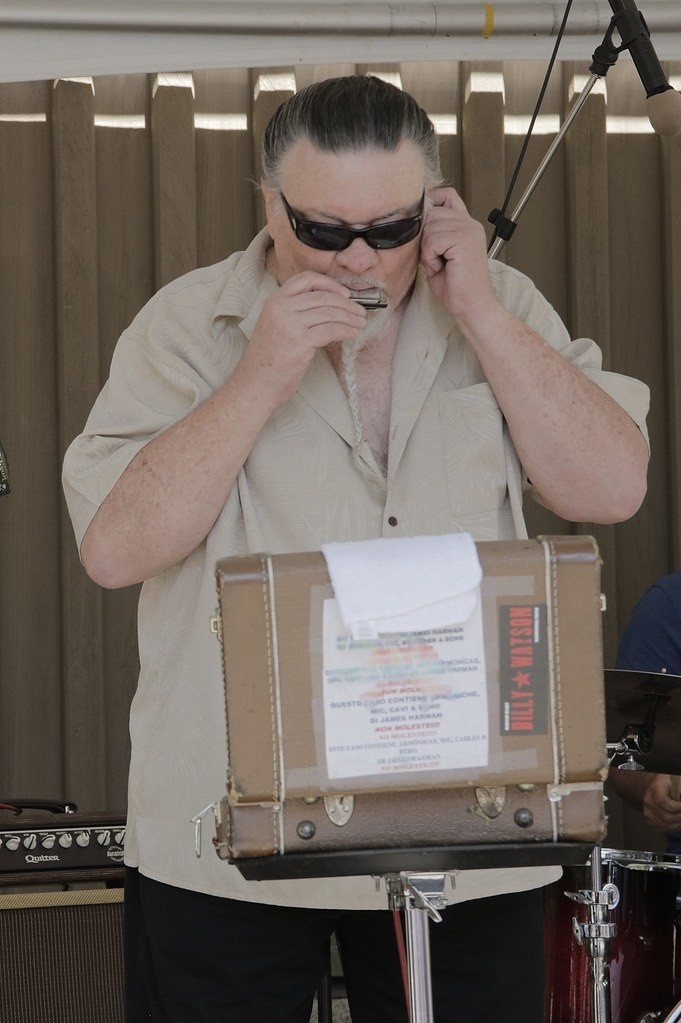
[608,570,681,855]
[57,74,651,1023]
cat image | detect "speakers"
[0,888,127,1023]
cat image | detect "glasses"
[280,188,425,251]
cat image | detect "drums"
[537,844,681,1023]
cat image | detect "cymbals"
[603,667,681,776]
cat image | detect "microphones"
[609,0,681,138]
